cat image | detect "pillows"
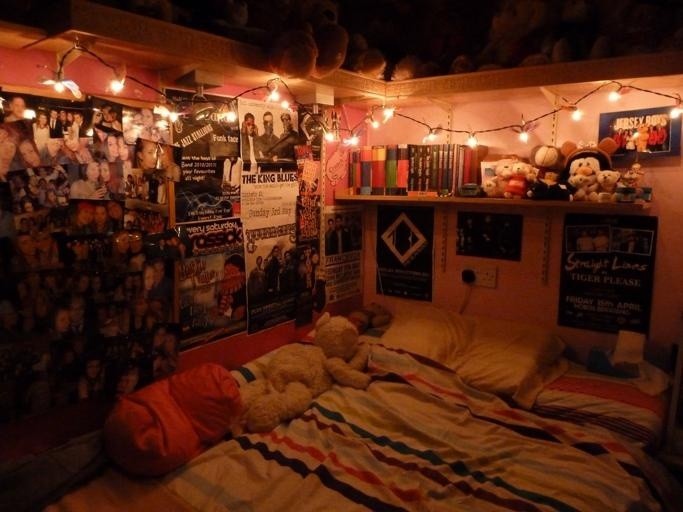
[105,363,243,477]
[457,320,559,396]
[372,304,465,366]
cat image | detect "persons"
[593,230,609,251]
[576,230,594,251]
[2,94,352,399]
[613,124,666,152]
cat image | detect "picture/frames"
[600,106,680,166]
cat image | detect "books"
[348,144,474,195]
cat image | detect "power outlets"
[460,265,496,288]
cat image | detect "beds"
[41,322,683,512]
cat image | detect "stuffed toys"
[129,0,683,81]
[230,312,371,438]
[483,138,623,202]
[346,302,390,335]
